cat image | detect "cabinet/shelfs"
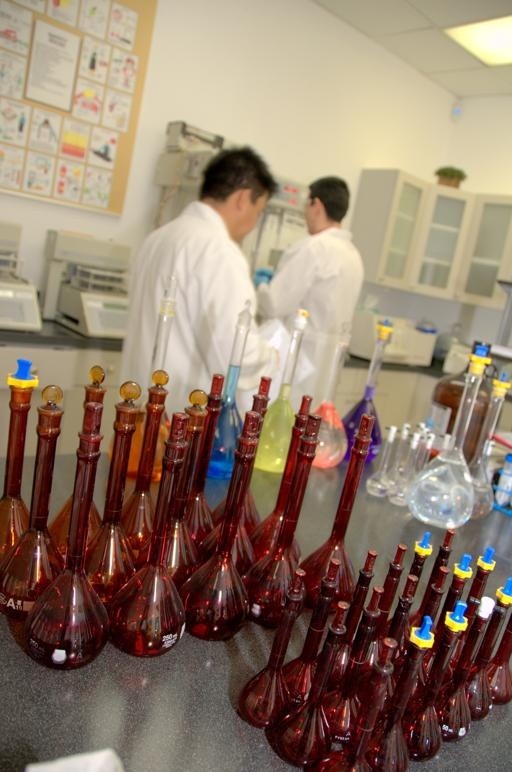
[334,368,420,439]
[58,348,125,456]
[350,169,476,301]
[452,193,511,313]
[0,342,77,457]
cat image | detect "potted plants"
[435,166,467,188]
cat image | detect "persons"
[253,177,367,418]
[120,148,291,421]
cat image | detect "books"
[238,318,314,414]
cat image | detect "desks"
[0,437,512,772]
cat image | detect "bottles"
[234,528,512,770]
[405,321,511,527]
[107,297,179,480]
[341,315,394,469]
[315,319,355,469]
[205,301,259,478]
[1,358,377,670]
[366,420,450,505]
[252,310,309,472]
